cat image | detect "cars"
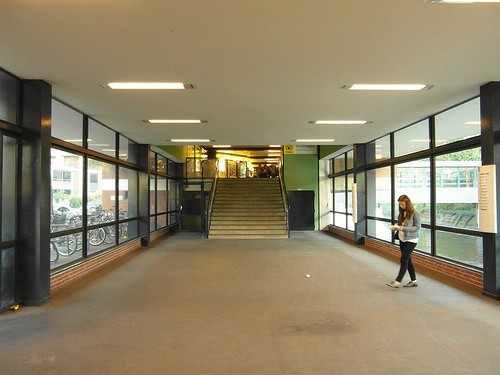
[52,204,77,224]
[79,201,103,212]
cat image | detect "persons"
[270,163,277,178]
[264,164,273,178]
[386,195,421,288]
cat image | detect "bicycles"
[50,206,129,263]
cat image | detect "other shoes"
[404,280,418,287]
[386,281,401,288]
